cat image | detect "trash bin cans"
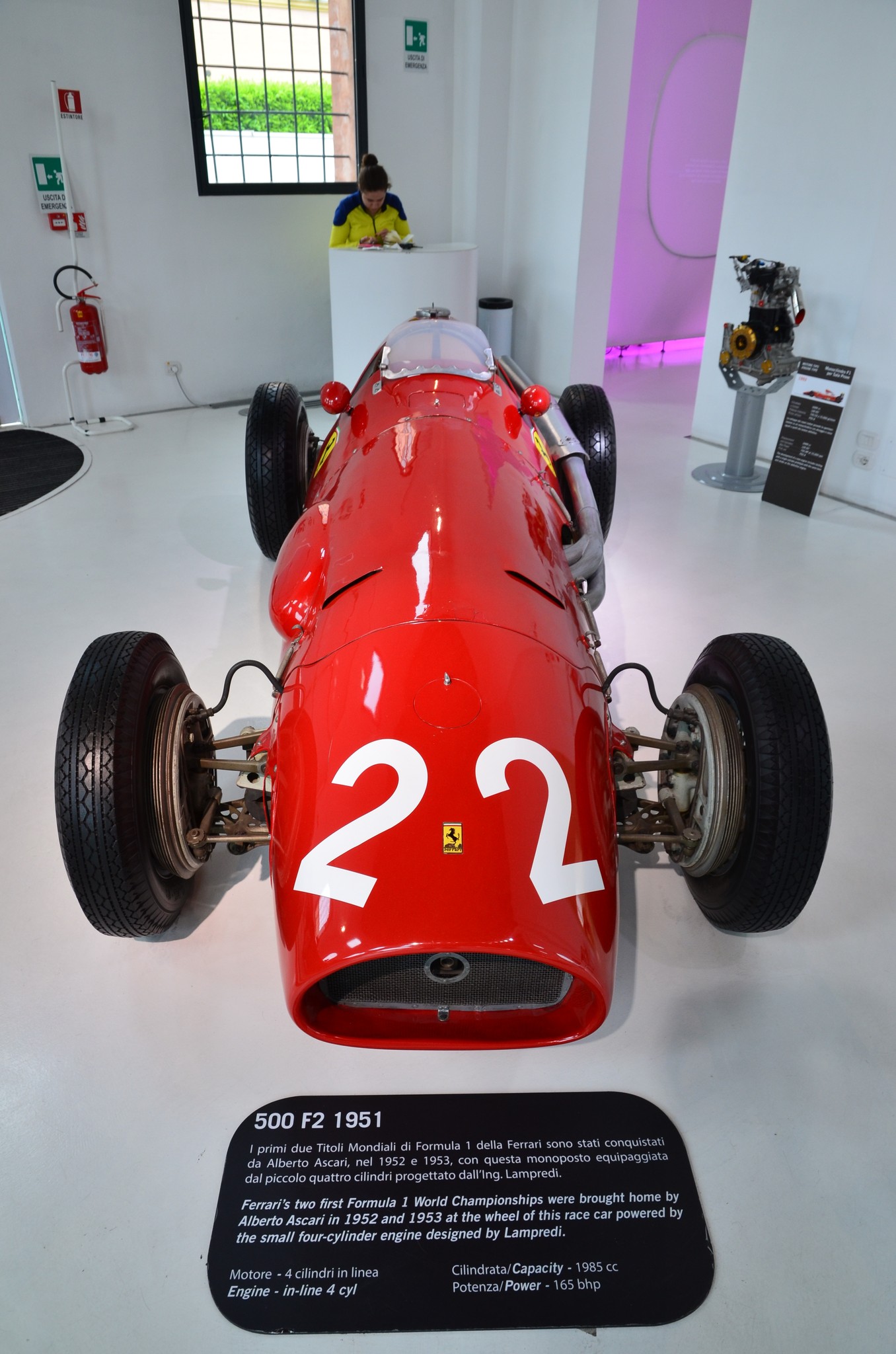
[476,297,513,360]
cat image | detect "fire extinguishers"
[52,265,110,375]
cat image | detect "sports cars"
[56,307,834,1051]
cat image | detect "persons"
[328,154,413,248]
